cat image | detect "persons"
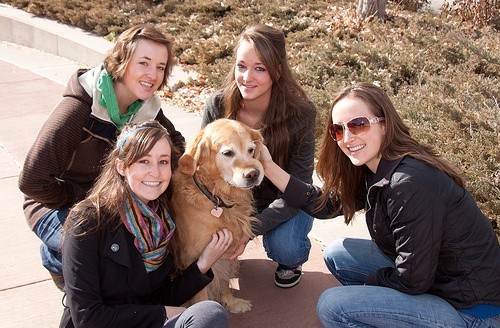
[201,25,318,288]
[18,23,187,294]
[255,82,500,328]
[59,119,230,328]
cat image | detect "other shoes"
[49,270,65,292]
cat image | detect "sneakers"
[274,263,302,288]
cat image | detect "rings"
[223,241,228,246]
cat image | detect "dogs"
[168,118,265,314]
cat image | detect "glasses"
[328,116,384,141]
